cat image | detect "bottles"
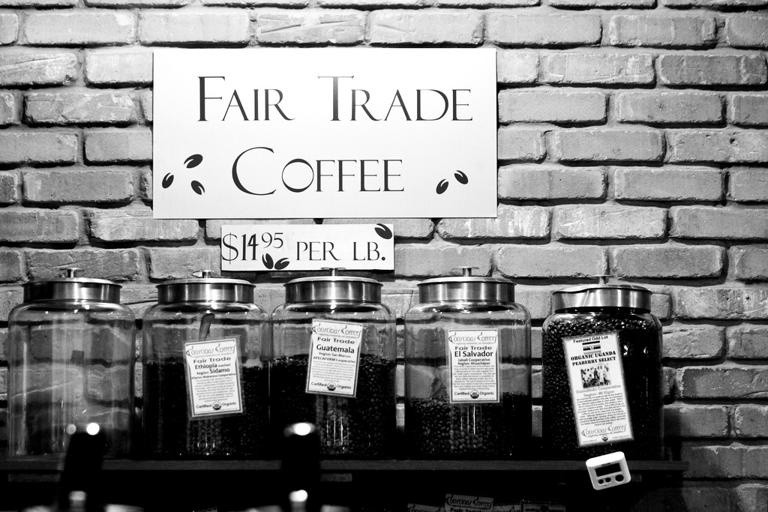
[4,269,136,462]
[270,275,398,458]
[540,283,665,464]
[141,272,268,458]
[404,269,532,458]
